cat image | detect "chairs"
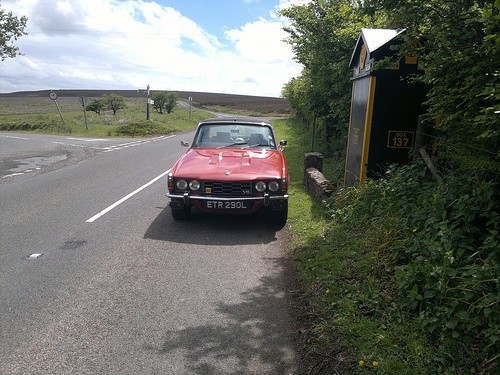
[213,131,234,143]
[245,133,267,144]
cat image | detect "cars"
[165,118,291,231]
